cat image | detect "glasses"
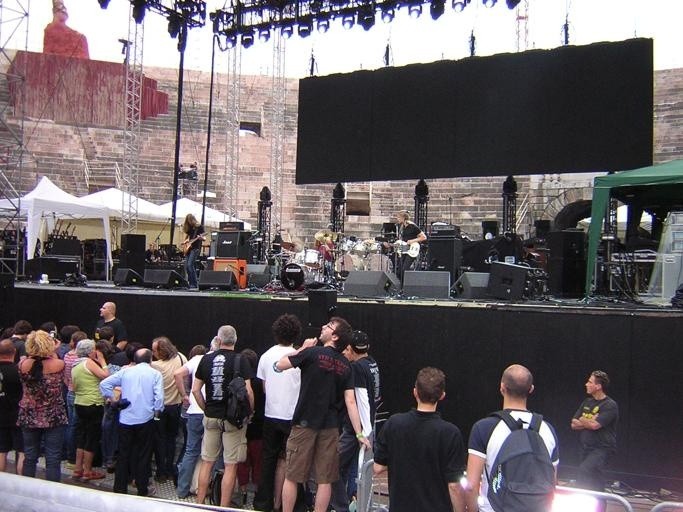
[326,324,335,330]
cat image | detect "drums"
[365,253,393,271]
[336,255,364,271]
[281,263,320,290]
[319,237,349,262]
[302,250,319,264]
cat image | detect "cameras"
[48,331,56,340]
[119,398,130,409]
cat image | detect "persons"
[0,235,17,273]
[571,369,619,512]
[145,242,161,264]
[319,234,335,280]
[1,303,381,512]
[180,213,206,292]
[372,365,467,511]
[464,363,560,511]
[395,209,428,296]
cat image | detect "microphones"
[57,220,63,235]
[383,222,396,238]
[63,222,71,235]
[168,217,174,220]
[69,226,76,239]
[53,219,59,230]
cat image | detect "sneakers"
[65,460,189,498]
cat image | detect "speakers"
[25,254,80,283]
[308,289,337,329]
[1,273,14,299]
[451,272,490,298]
[198,270,239,291]
[534,220,550,237]
[550,230,586,298]
[486,261,546,299]
[113,269,143,285]
[121,234,145,278]
[344,271,395,297]
[220,222,244,231]
[216,230,251,260]
[481,221,499,238]
[247,265,272,287]
[428,238,463,285]
[143,269,188,288]
[402,271,451,299]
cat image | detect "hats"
[351,330,368,350]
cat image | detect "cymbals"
[281,241,302,252]
[315,230,337,243]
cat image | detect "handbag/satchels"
[226,370,250,429]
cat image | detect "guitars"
[179,232,208,256]
[384,240,420,258]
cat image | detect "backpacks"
[485,410,557,512]
[208,469,244,508]
